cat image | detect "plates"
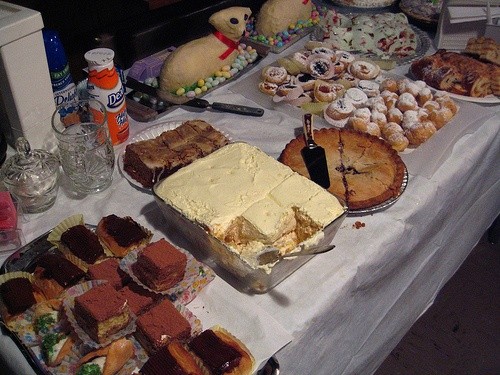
[310,22,431,66]
[410,61,500,104]
[118,120,236,192]
[0,223,280,375]
[345,163,408,215]
[321,0,400,16]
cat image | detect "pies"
[278,128,404,210]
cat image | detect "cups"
[50,97,115,194]
[0,136,62,214]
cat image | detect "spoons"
[258,244,335,265]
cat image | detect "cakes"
[157,6,252,97]
[255,0,316,37]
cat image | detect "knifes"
[125,80,264,117]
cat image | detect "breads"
[121,119,229,188]
[409,37,500,99]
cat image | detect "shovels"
[296,109,332,191]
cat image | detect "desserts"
[324,12,418,54]
[259,40,458,151]
[0,213,256,375]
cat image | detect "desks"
[0,33,500,375]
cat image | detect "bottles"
[84,47,130,147]
[43,32,84,126]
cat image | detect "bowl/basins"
[151,139,350,294]
